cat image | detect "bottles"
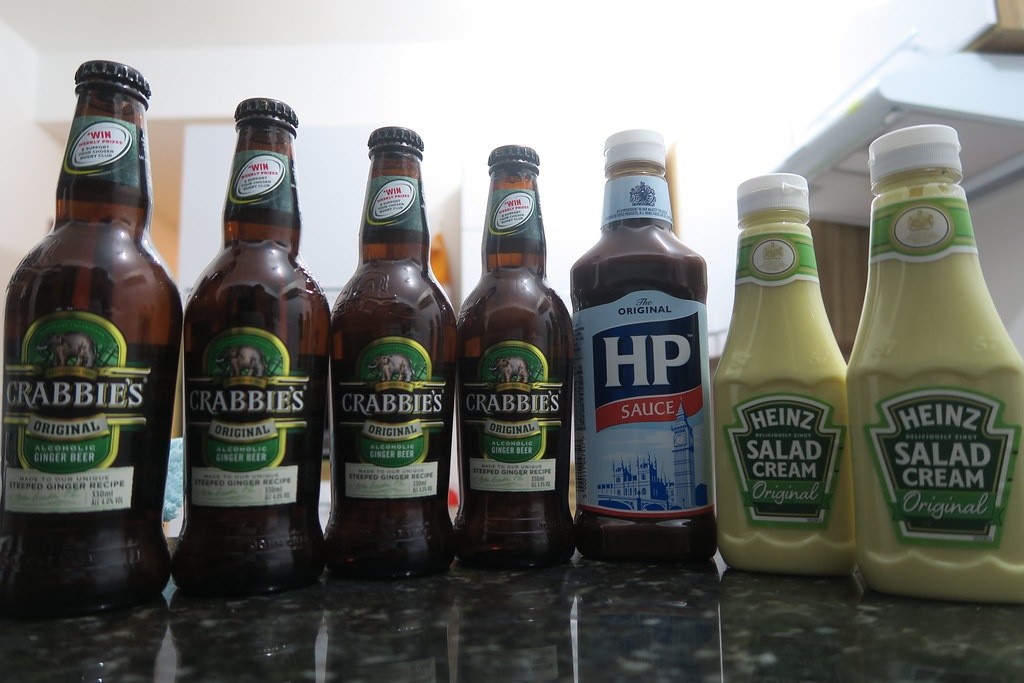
[3,577,1024,683]
[1,59,1024,617]
